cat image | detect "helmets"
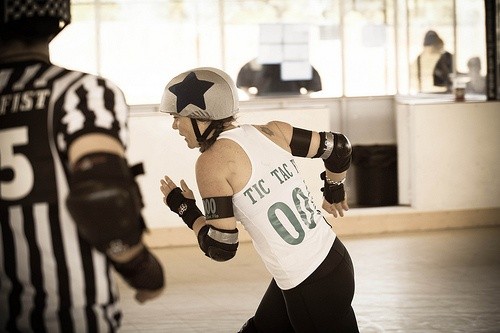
[0,0,72,44]
[161,66,239,120]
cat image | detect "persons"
[410,30,454,95]
[465,56,487,95]
[158,67,360,333]
[0,0,165,333]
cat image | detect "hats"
[425,31,438,45]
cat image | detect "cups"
[454,84,466,102]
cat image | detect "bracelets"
[326,175,347,186]
